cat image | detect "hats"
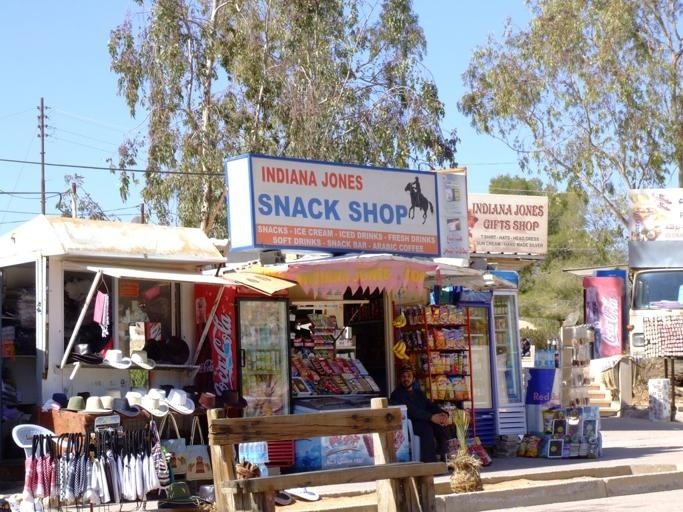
[157,482,200,505]
[69,344,157,370]
[52,388,247,417]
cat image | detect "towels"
[93,291,105,324]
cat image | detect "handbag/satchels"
[184,416,212,483]
[158,411,187,476]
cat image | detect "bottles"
[563,340,591,408]
[193,294,215,436]
[239,297,283,398]
[534,337,564,369]
[585,286,603,359]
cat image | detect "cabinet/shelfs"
[392,301,475,437]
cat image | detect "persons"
[521,339,530,356]
[389,369,450,464]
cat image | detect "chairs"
[12,424,68,459]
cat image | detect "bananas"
[392,311,406,328]
[393,340,410,360]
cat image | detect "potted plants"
[448,409,483,493]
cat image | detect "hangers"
[96,270,109,296]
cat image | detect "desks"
[33,406,194,455]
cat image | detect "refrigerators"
[191,277,296,469]
[437,284,497,456]
[280,393,412,474]
[481,270,529,437]
[580,268,628,359]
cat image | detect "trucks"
[626,239,682,368]
